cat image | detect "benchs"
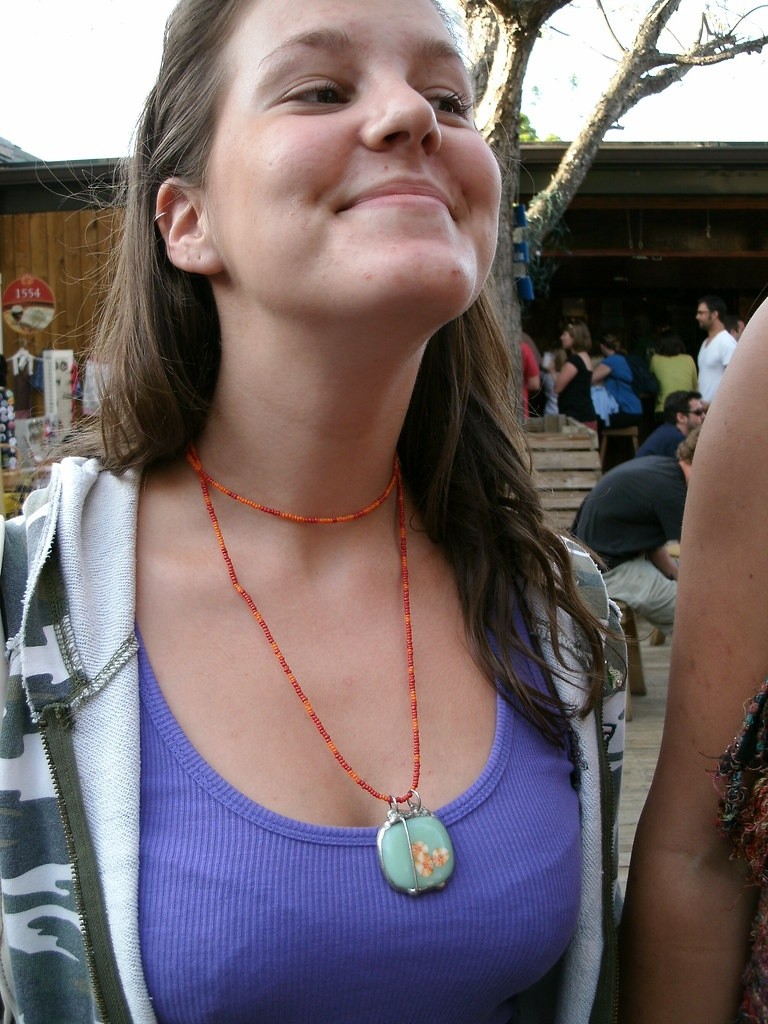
[525,432,650,723]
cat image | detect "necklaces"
[188,452,455,894]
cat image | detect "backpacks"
[609,351,659,398]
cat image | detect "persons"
[616,298,768,1024]
[521,298,746,434]
[571,423,701,638]
[0,0,629,1023]
[636,391,706,459]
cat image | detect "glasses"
[696,311,716,316]
[679,408,704,416]
